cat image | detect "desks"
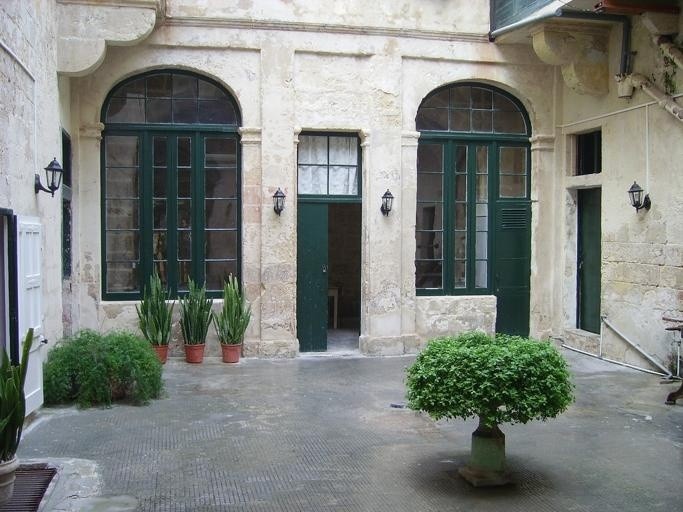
[328,286,340,329]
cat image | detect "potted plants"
[0,325,35,507]
[403,330,579,489]
[69,327,170,411]
[135,271,252,364]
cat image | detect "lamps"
[380,189,394,217]
[35,156,65,198]
[272,187,286,217]
[627,180,652,213]
